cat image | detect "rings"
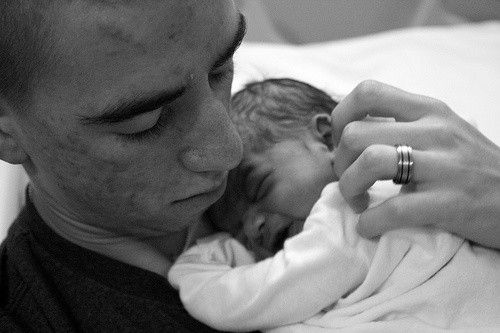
[394,142,414,184]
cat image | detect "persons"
[0,0,500,333]
[166,79,500,333]
